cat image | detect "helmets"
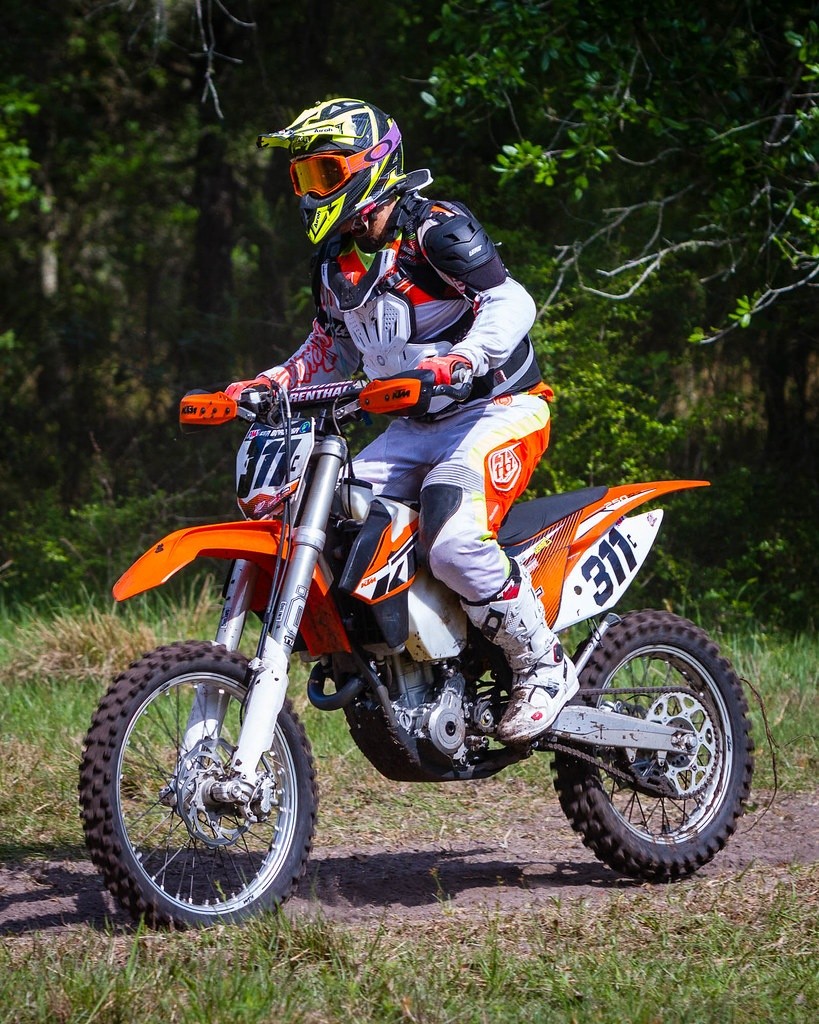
[256,97,406,245]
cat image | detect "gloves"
[418,355,470,392]
[224,377,275,410]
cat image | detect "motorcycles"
[79,364,756,932]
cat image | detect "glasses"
[290,154,351,196]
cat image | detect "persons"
[221,96,583,744]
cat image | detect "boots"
[458,556,582,746]
